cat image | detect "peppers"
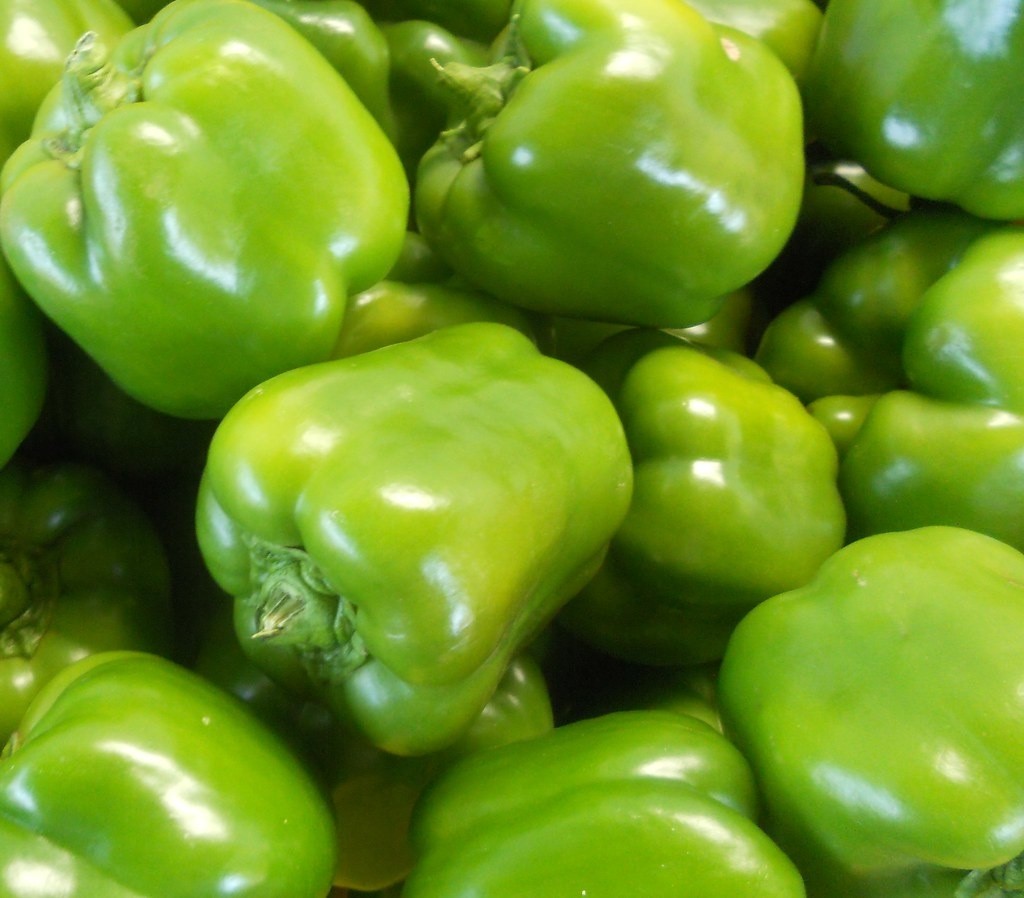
[0,0,1024,897]
